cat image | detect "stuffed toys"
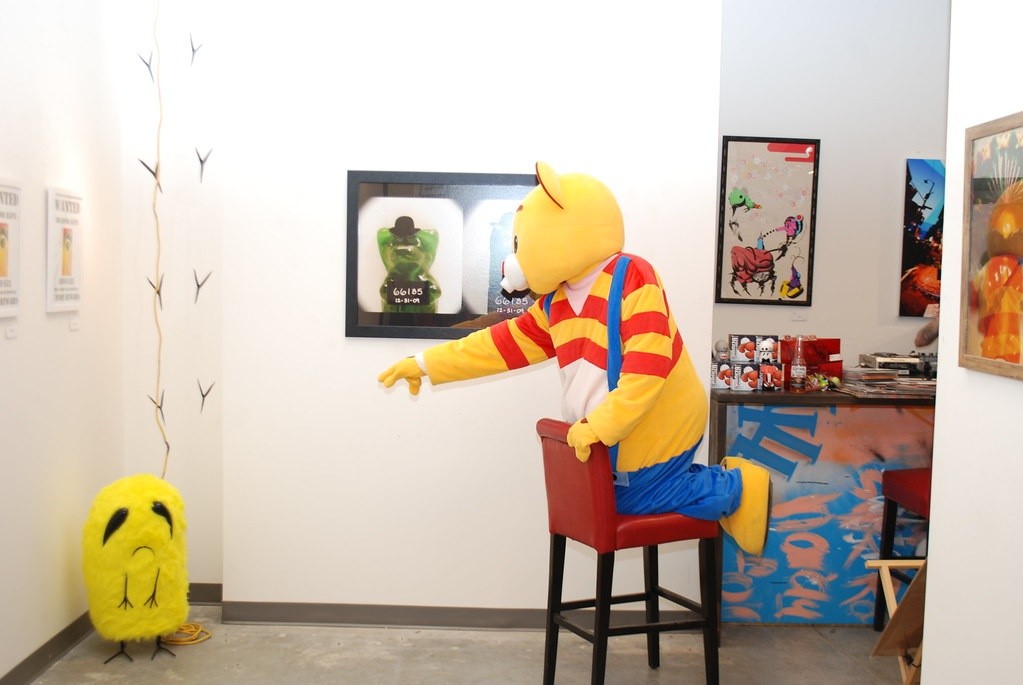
[378,161,772,555]
[969,179,1023,363]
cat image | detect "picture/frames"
[47,188,85,314]
[714,135,820,307]
[0,182,23,317]
[345,170,540,340]
[958,112,1023,381]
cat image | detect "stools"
[874,465,933,634]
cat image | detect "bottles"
[790,335,806,394]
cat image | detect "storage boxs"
[711,332,842,392]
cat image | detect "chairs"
[535,417,720,684]
